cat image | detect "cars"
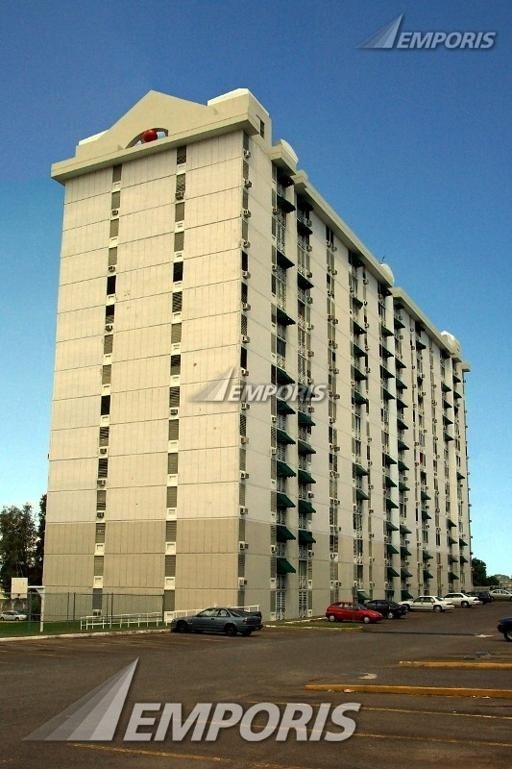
[465,591,491,605]
[489,588,512,601]
[364,600,408,619]
[397,596,456,613]
[496,615,512,642]
[171,607,263,636]
[0,609,28,621]
[326,601,384,624]
[439,593,483,609]
